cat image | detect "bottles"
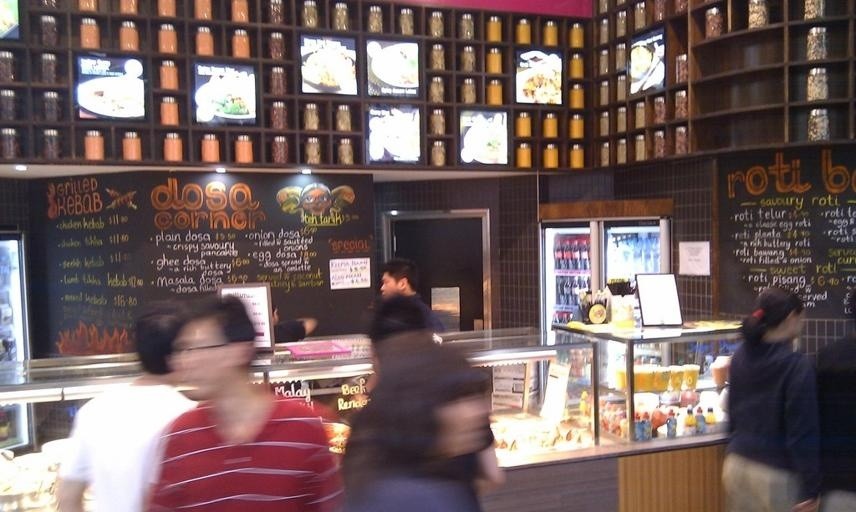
[1,1,587,169]
[600,80,608,105]
[608,237,661,275]
[804,1,826,20]
[615,1,626,5]
[616,138,627,164]
[599,49,608,74]
[554,234,593,379]
[807,68,829,101]
[617,75,626,100]
[705,8,724,37]
[748,1,769,28]
[655,1,665,22]
[600,1,607,13]
[653,96,666,124]
[617,107,626,132]
[653,130,664,158]
[634,3,646,30]
[632,343,663,363]
[676,127,687,154]
[675,0,687,14]
[808,109,828,141]
[600,19,609,43]
[576,391,716,440]
[807,27,829,61]
[600,142,609,166]
[600,112,610,136]
[615,44,625,70]
[675,54,688,83]
[0,406,20,442]
[635,134,645,161]
[635,101,645,128]
[616,11,626,37]
[675,90,688,120]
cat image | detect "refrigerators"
[540,215,671,399]
[0,227,35,451]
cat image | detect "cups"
[709,355,730,386]
[616,363,699,393]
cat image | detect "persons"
[342,296,507,500]
[340,343,491,512]
[813,285,856,512]
[721,286,822,512]
[145,293,347,512]
[56,303,202,512]
[271,303,319,344]
[380,259,444,335]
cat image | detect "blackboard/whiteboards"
[711,145,856,316]
[28,170,374,368]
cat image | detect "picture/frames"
[634,271,685,328]
[215,281,276,354]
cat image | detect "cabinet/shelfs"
[0,324,602,511]
[0,0,855,181]
[551,315,803,443]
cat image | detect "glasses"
[172,340,231,355]
